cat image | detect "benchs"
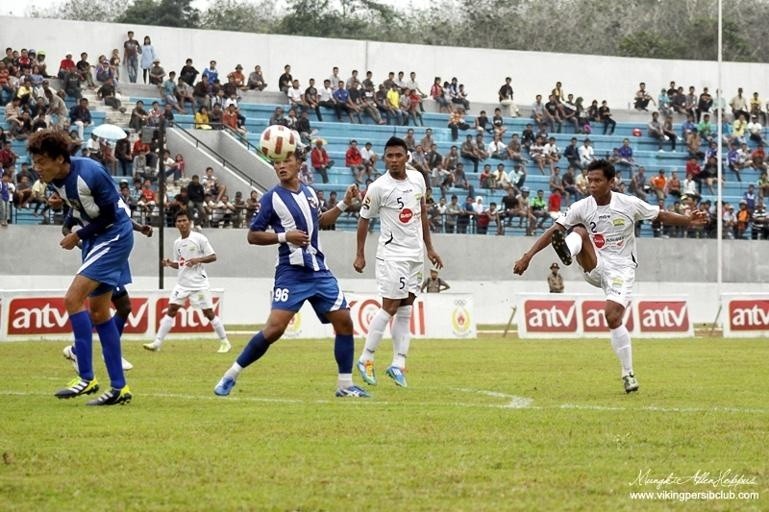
[0,92,769,242]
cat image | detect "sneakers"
[384,365,408,388]
[214,369,237,397]
[101,351,134,371]
[142,342,161,352]
[216,342,232,353]
[334,386,372,399]
[356,360,376,386]
[84,384,134,407]
[622,371,636,394]
[54,374,100,399]
[61,342,80,375]
[551,230,572,267]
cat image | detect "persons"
[353,134,444,389]
[348,70,767,240]
[548,262,564,293]
[420,267,450,292]
[27,130,135,407]
[0,25,380,229]
[513,160,708,391]
[213,138,373,400]
[141,210,232,355]
[48,185,151,370]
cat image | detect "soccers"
[260,125,298,161]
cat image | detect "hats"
[429,268,438,275]
